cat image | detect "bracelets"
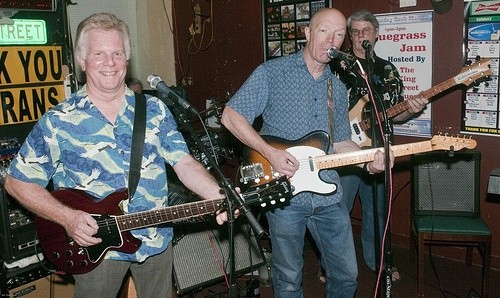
[363,162,374,175]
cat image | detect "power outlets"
[208,7,215,23]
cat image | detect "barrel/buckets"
[259,252,273,287]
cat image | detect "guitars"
[242,130,477,201]
[35,172,291,274]
[348,56,493,149]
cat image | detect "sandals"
[317,257,327,285]
[381,266,402,284]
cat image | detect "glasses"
[349,27,375,36]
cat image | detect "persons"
[4,13,241,298]
[318,10,430,284]
[221,8,395,298]
[125,78,143,94]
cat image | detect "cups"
[246,279,260,298]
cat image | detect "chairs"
[409,148,493,298]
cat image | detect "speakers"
[0,255,140,298]
[171,205,268,295]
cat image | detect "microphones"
[362,40,372,50]
[327,46,357,61]
[147,74,198,115]
